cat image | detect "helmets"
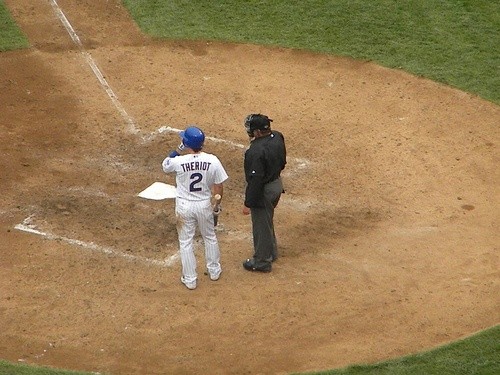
[180,126,205,149]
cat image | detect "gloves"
[213,204,221,215]
[177,142,185,150]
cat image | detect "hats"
[250,114,273,130]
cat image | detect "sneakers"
[243,259,271,272]
[210,269,222,280]
[181,276,196,289]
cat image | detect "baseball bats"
[213,193,222,227]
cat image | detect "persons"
[162,126,229,290]
[243,113,287,272]
